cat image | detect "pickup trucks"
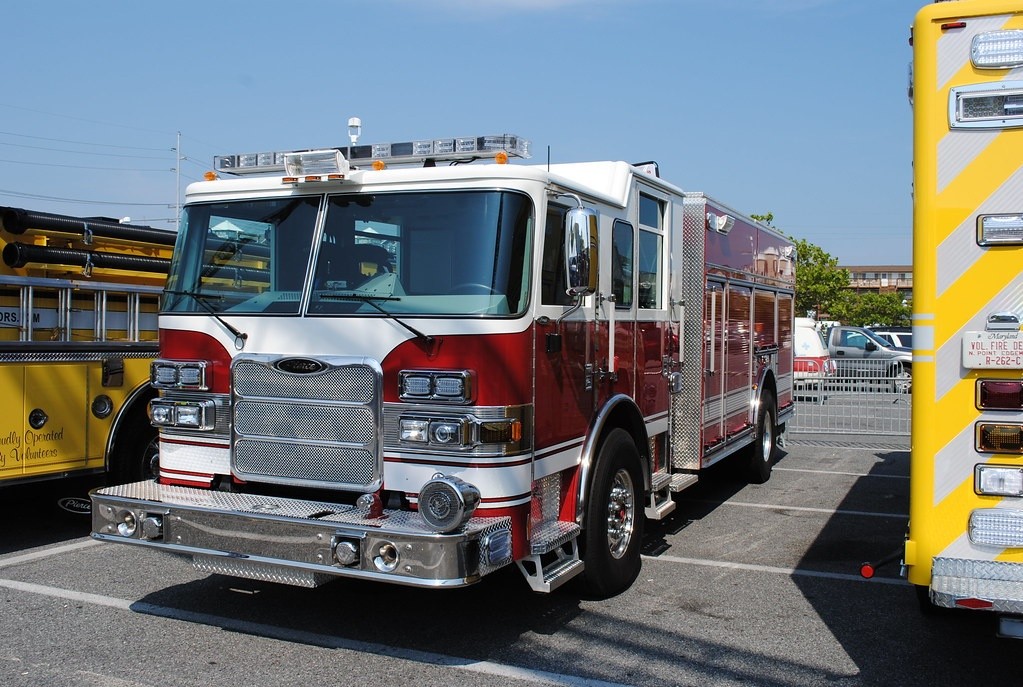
[824,324,912,395]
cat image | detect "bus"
[860,1,1023,621]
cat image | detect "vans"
[793,316,836,389]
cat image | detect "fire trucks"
[84,113,798,603]
[0,206,403,490]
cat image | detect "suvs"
[842,323,912,352]
